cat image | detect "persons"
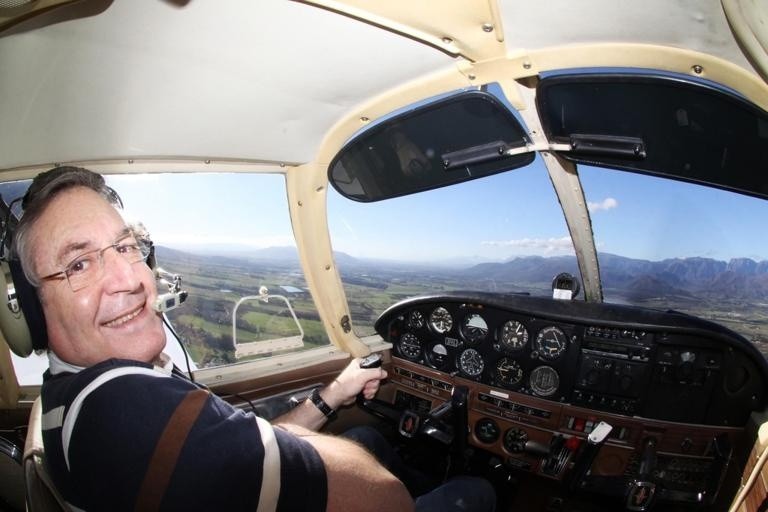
[11,165,415,511]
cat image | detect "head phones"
[0,166,157,358]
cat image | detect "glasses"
[39,230,151,292]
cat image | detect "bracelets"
[308,389,337,421]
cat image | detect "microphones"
[151,267,188,312]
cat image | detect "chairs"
[20,393,91,512]
